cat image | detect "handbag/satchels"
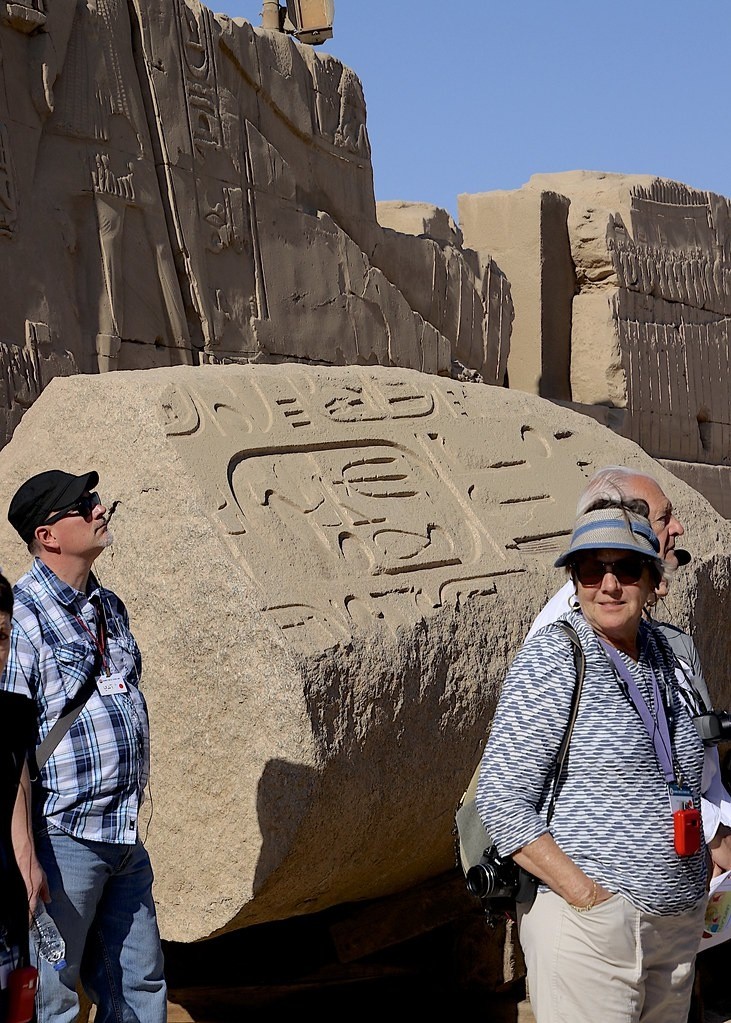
[455,757,494,881]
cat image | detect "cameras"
[465,846,520,899]
[691,710,731,748]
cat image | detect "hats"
[8,469,99,544]
[553,509,662,567]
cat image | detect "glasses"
[571,557,652,585]
[39,491,102,525]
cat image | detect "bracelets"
[569,879,598,912]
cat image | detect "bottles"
[26,893,67,971]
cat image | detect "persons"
[474,496,710,1023]
[521,466,731,1023]
[0,468,167,1023]
[607,236,731,316]
